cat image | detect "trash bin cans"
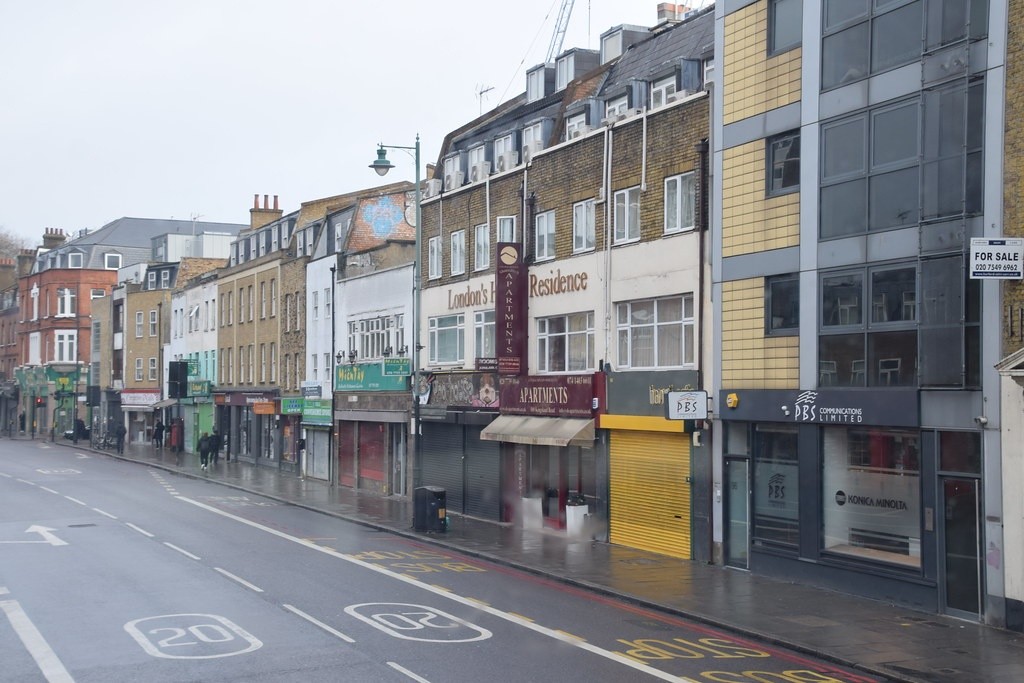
[411,484,447,534]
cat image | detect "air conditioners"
[425,179,441,196]
[522,141,543,165]
[667,89,686,103]
[601,113,623,126]
[471,162,490,181]
[617,108,643,120]
[497,151,518,171]
[571,124,595,137]
[444,172,463,191]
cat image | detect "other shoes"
[201,464,208,474]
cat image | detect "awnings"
[480,414,596,448]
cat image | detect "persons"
[76,417,86,444]
[115,420,127,454]
[197,431,210,471]
[152,419,165,448]
[209,430,220,464]
[18,407,26,433]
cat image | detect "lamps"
[349,350,357,363]
[397,345,406,357]
[383,347,391,357]
[335,350,345,364]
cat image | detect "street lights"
[365,132,425,505]
